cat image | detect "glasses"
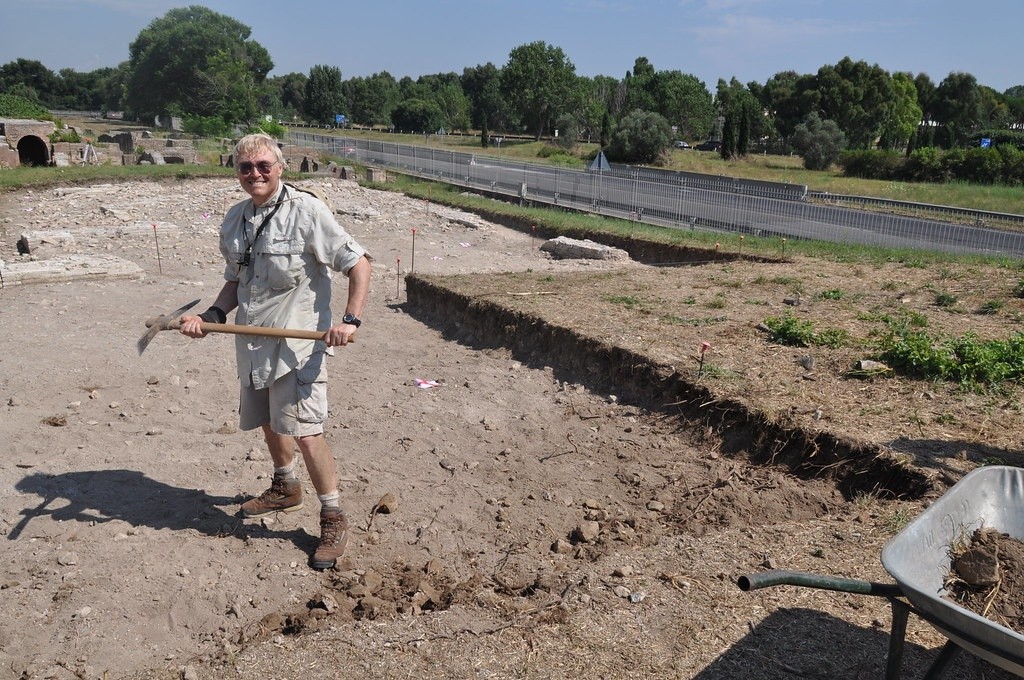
[236,159,280,175]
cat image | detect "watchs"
[342,312,361,328]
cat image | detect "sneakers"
[311,506,349,570]
[240,478,304,518]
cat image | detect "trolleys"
[737,465,1024,679]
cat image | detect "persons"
[180,134,372,568]
